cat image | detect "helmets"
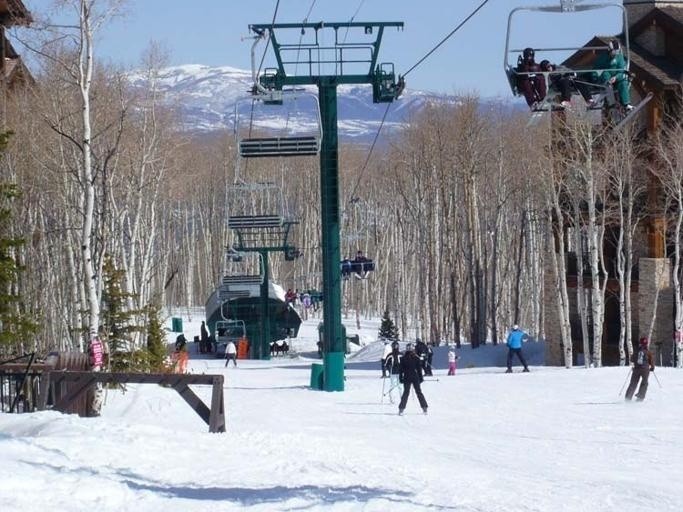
[639,337,648,346]
[512,324,520,331]
[391,342,400,352]
[406,342,416,352]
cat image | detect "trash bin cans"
[172,317,183,332]
[317,322,346,358]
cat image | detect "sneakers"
[505,367,513,373]
[398,407,404,413]
[423,406,429,412]
[522,368,530,372]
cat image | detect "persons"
[423,342,432,376]
[504,324,529,372]
[382,341,402,405]
[446,345,460,375]
[621,337,655,401]
[283,286,325,311]
[540,59,600,110]
[589,38,636,112]
[381,340,392,378]
[339,256,351,281]
[511,48,566,113]
[413,338,427,369]
[223,340,237,368]
[175,333,186,353]
[396,343,429,413]
[352,250,371,281]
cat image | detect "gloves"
[399,378,404,383]
[649,368,654,371]
[418,378,424,383]
